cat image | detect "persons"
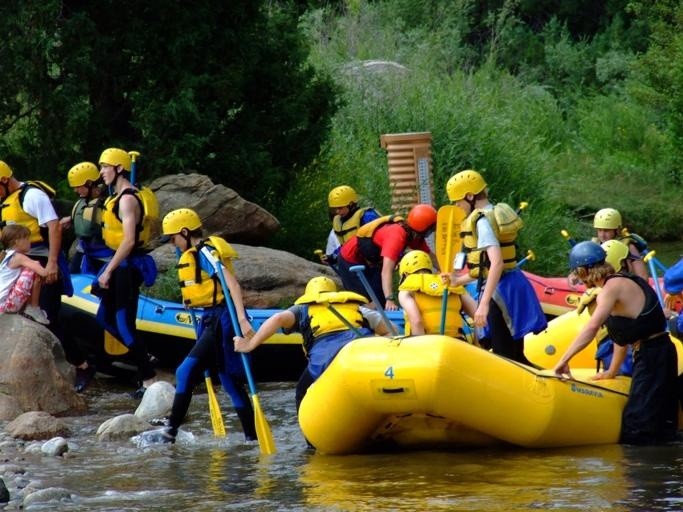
[0,223,51,327]
[136,207,259,443]
[586,239,634,379]
[661,258,683,345]
[58,161,105,275]
[231,276,396,451]
[316,168,549,369]
[551,240,681,443]
[0,159,98,393]
[92,147,157,400]
[592,206,649,283]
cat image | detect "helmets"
[98,147,132,171]
[0,160,13,182]
[328,185,359,208]
[162,207,202,236]
[599,239,629,274]
[305,276,336,295]
[445,169,488,206]
[407,203,438,232]
[67,162,100,188]
[592,207,624,231]
[568,241,606,270]
[398,249,433,285]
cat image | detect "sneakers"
[24,303,51,324]
[140,429,176,445]
[133,384,148,401]
[74,365,95,393]
[147,353,159,367]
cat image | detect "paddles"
[173,246,227,439]
[435,204,467,336]
[209,250,275,458]
[643,250,683,377]
